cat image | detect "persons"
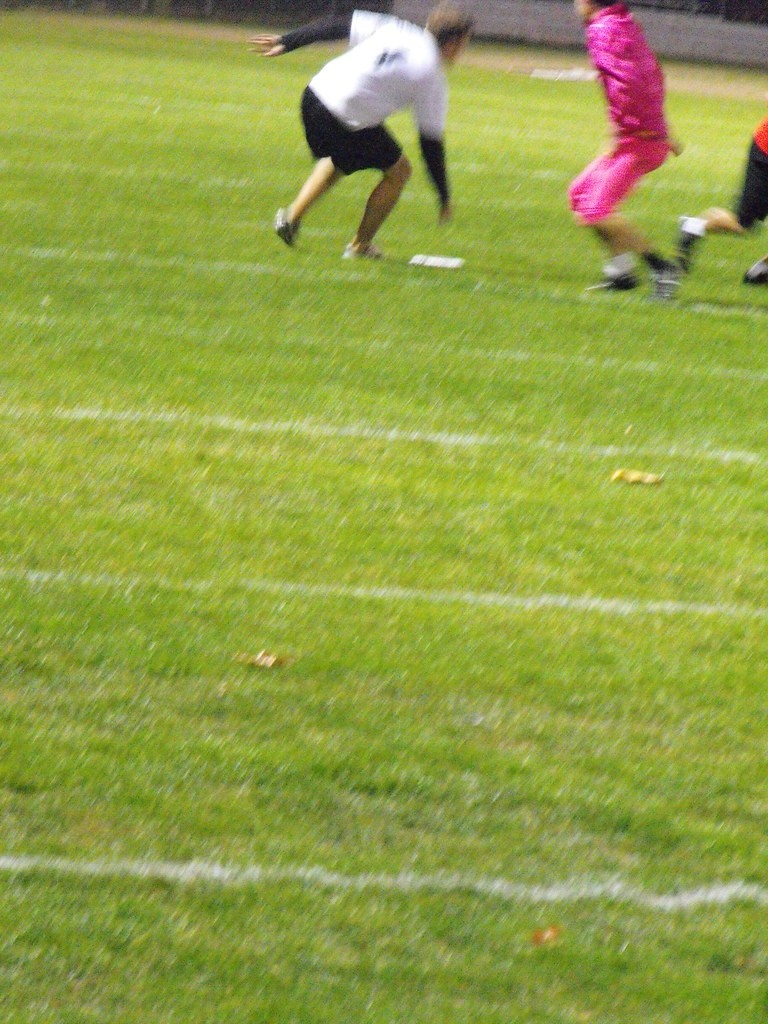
[247,3,477,260]
[669,118,768,287]
[566,0,684,302]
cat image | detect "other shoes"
[585,272,640,293]
[676,216,703,272]
[343,244,381,260]
[744,266,768,284]
[276,219,297,244]
[651,262,685,308]
